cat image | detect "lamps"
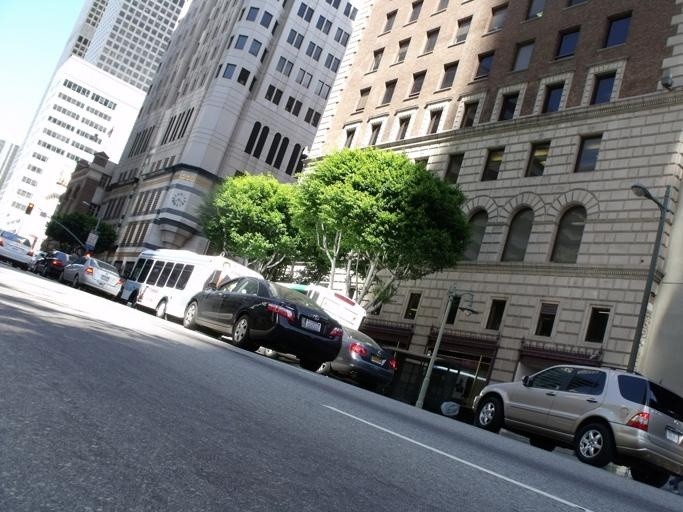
[661,70,674,91]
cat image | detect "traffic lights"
[25,202,35,215]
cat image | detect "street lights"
[81,200,103,254]
[626,183,670,372]
[415,287,479,408]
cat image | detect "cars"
[0,229,122,300]
[472,364,683,488]
[183,276,400,394]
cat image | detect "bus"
[120,249,265,320]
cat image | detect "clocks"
[171,192,187,207]
